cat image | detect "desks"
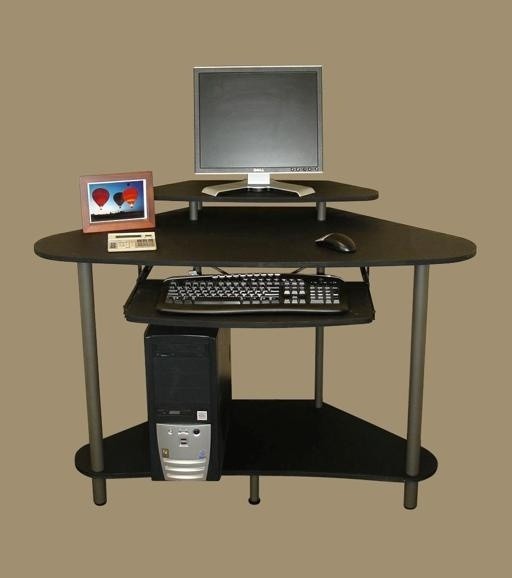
[33,180,476,510]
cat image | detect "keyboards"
[156,273,349,314]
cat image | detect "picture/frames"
[81,172,156,233]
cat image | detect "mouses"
[315,233,356,252]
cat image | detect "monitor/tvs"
[193,65,323,197]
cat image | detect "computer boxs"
[144,323,232,481]
